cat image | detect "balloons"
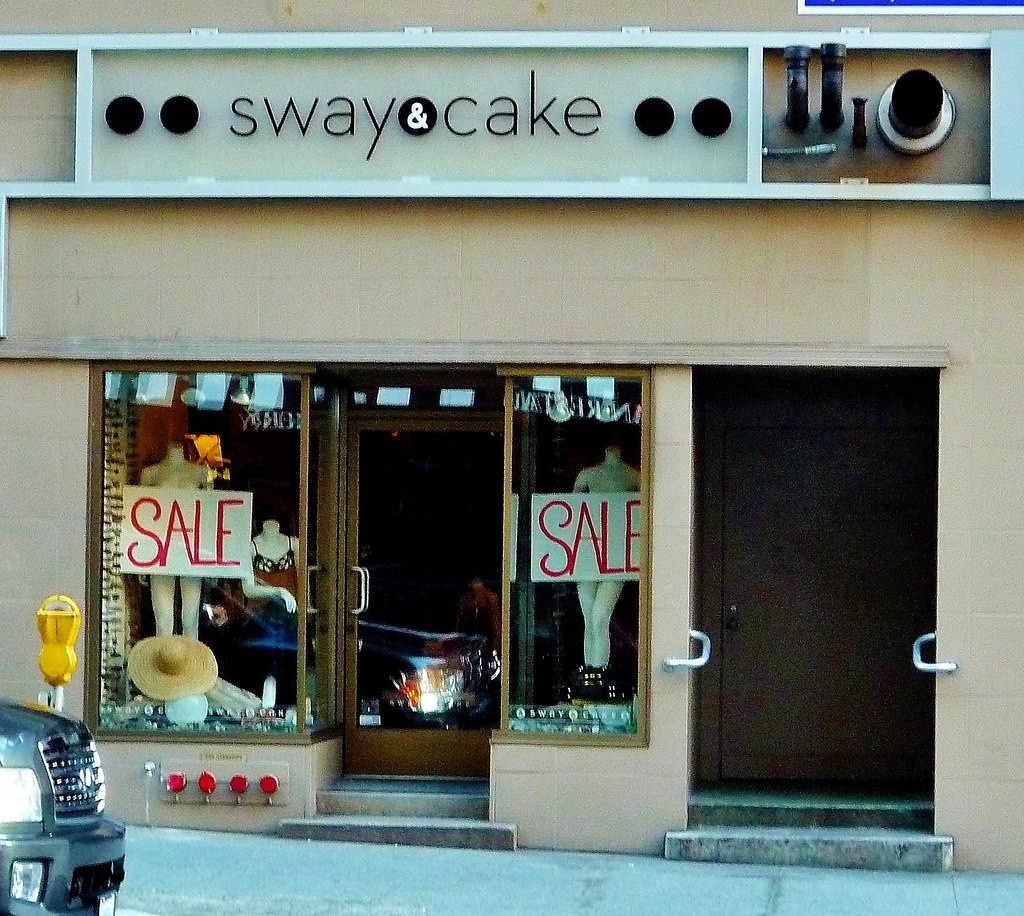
[163,693,210,727]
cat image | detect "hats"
[127,635,219,701]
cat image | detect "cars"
[198,573,494,730]
[0,699,126,916]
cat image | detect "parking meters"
[37,596,85,718]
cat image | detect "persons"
[457,580,502,658]
[572,447,642,668]
[137,444,217,643]
[240,521,299,622]
